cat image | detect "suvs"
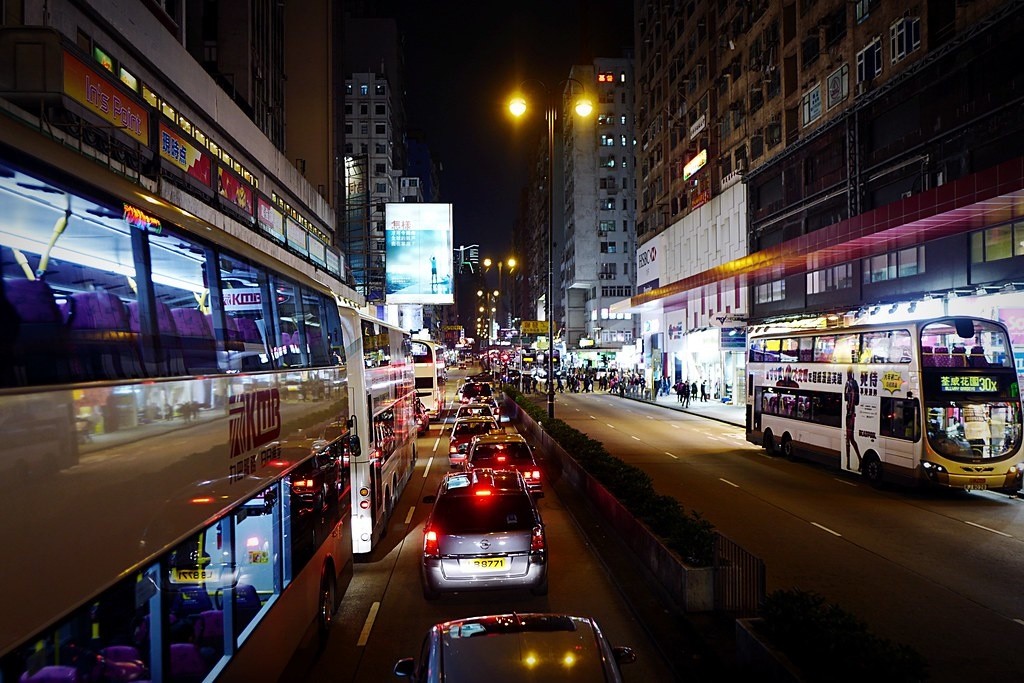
[446,414,506,469]
[418,467,550,605]
[457,432,544,500]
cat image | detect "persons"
[522,364,707,408]
[797,403,806,418]
[844,366,863,472]
[691,381,697,401]
[429,255,437,284]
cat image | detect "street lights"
[516,74,595,419]
[482,256,516,381]
[476,286,501,375]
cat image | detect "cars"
[464,369,504,383]
[452,380,501,431]
[393,611,637,683]
[499,368,521,387]
[415,400,432,436]
[458,353,480,370]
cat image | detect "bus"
[335,302,421,554]
[412,338,452,422]
[0,119,354,683]
[743,313,1024,492]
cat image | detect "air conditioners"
[685,180,698,190]
[599,274,606,280]
[374,172,381,177]
[607,274,614,280]
[737,159,747,171]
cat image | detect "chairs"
[754,349,811,362]
[20,586,261,683]
[922,346,988,367]
[0,279,325,390]
[763,397,840,419]
